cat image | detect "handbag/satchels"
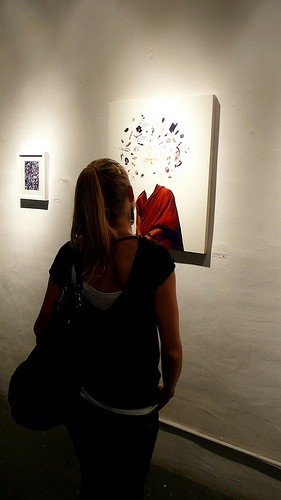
[8,241,87,432]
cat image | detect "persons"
[33,159,183,500]
